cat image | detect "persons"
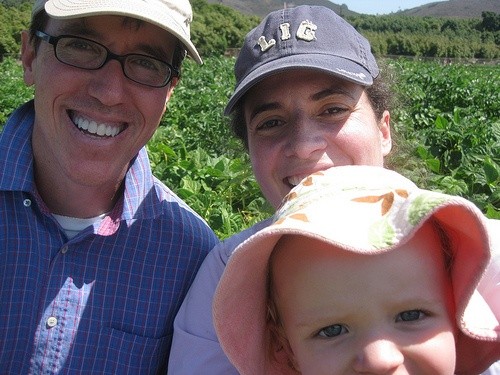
[0,0,220,375]
[168,6,499,374]
[207,165,500,375]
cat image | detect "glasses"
[35,29,181,87]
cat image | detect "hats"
[30,0,203,66]
[223,5,381,119]
[212,164,500,375]
